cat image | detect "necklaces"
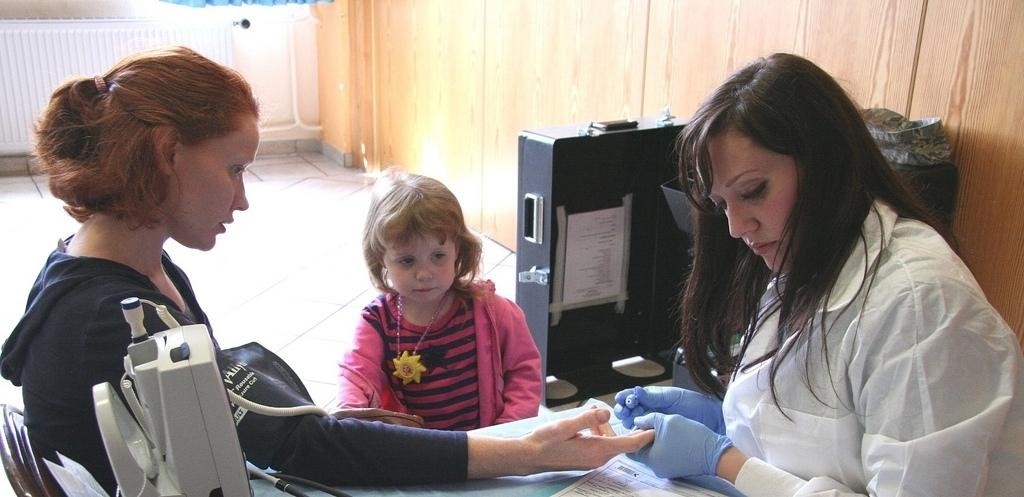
[391,293,449,381]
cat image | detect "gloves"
[613,383,735,479]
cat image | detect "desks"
[240,401,721,497]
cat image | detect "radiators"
[1,20,231,158]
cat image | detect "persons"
[613,47,1023,495]
[337,168,543,429]
[1,47,656,495]
